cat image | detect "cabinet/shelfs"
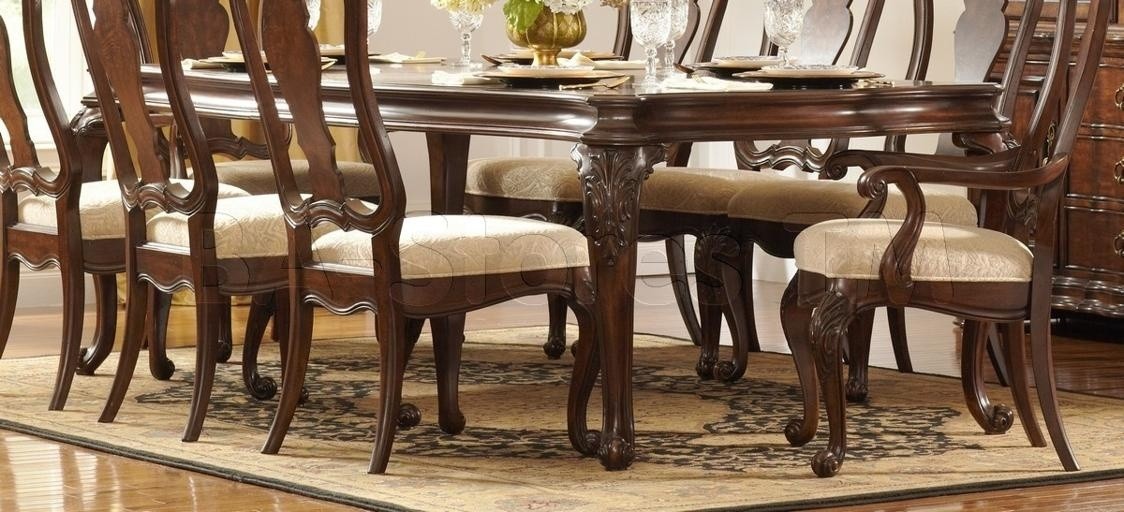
[967,0,1124,319]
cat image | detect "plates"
[474,51,625,84]
[201,49,382,73]
[695,56,886,87]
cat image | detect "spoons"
[673,60,695,75]
[559,76,630,91]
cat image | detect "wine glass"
[762,1,814,66]
[630,0,672,89]
[449,10,484,64]
[664,0,689,81]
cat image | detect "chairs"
[0,0,1113,475]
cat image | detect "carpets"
[0,323,1123,512]
